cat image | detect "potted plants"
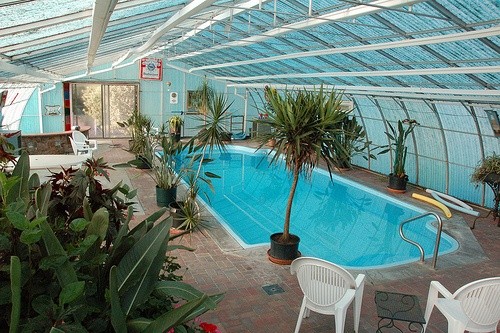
[470,151,500,191]
[244,83,382,260]
[377,118,420,190]
[108,75,242,247]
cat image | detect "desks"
[374,290,426,333]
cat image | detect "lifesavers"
[425,188,473,211]
[412,192,452,218]
[430,191,480,217]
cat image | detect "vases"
[266,139,276,146]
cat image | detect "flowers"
[0,93,228,333]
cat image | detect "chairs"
[290,257,366,333]
[422,277,500,333]
[69,130,98,155]
